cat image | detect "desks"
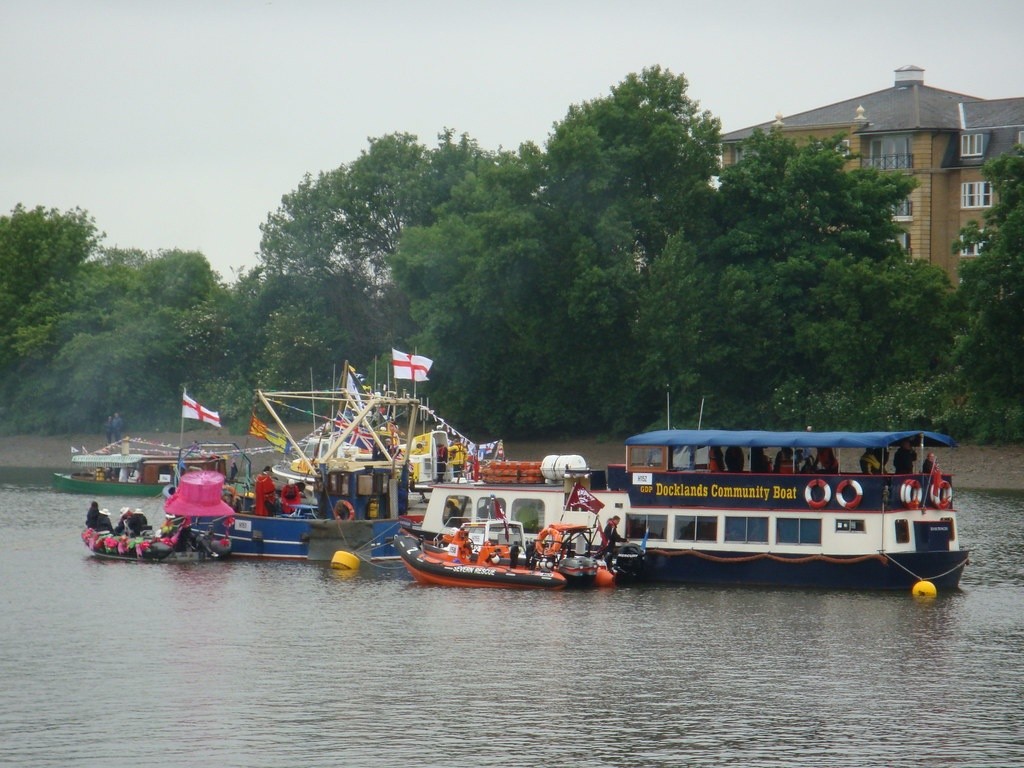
[289,504,319,519]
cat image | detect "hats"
[120,507,129,516]
[263,465,272,472]
[99,509,111,515]
[297,482,305,493]
[133,508,144,514]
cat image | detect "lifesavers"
[483,460,543,484]
[804,479,832,508]
[900,478,923,509]
[930,479,952,509]
[836,479,864,509]
[535,527,562,556]
[333,499,354,522]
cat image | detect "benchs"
[282,512,317,519]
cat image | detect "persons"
[707,440,935,473]
[86,461,303,537]
[372,437,542,536]
[104,413,123,443]
[601,516,629,572]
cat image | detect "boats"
[80,527,230,561]
[392,531,567,593]
[51,437,243,499]
[439,529,638,592]
[397,429,969,592]
[173,390,510,561]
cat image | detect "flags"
[250,415,290,455]
[569,484,604,515]
[182,394,220,428]
[346,374,363,411]
[492,495,510,543]
[393,348,434,382]
[333,412,373,451]
